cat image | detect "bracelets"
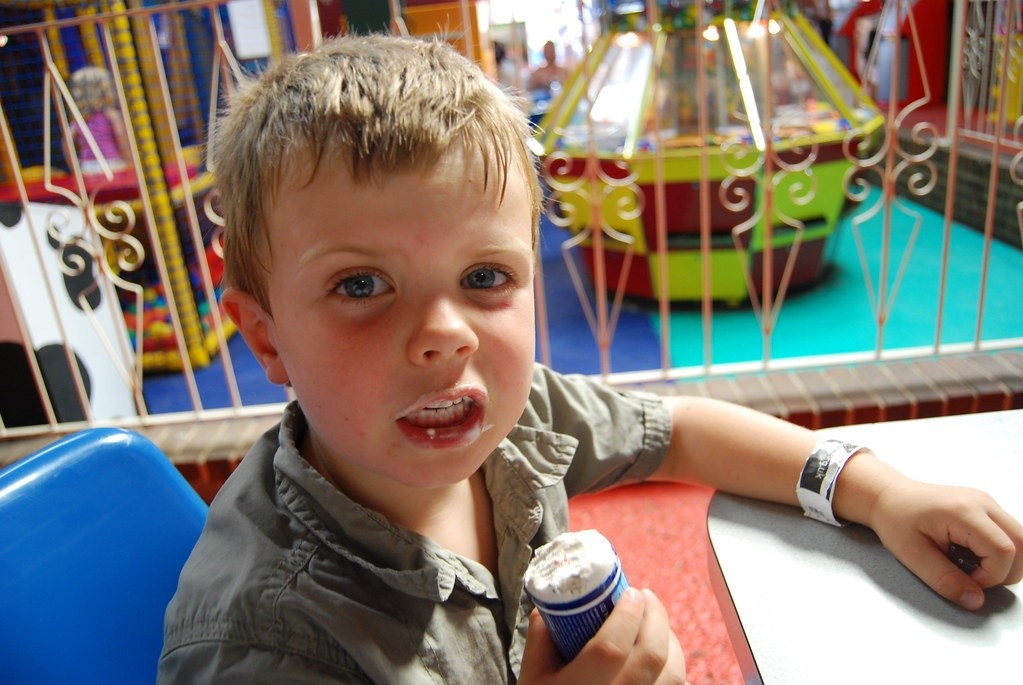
[796,439,874,529]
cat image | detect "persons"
[156,32,1023,685]
[524,40,569,89]
[62,67,131,174]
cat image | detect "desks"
[707,409,1023,685]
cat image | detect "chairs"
[0,427,210,684]
